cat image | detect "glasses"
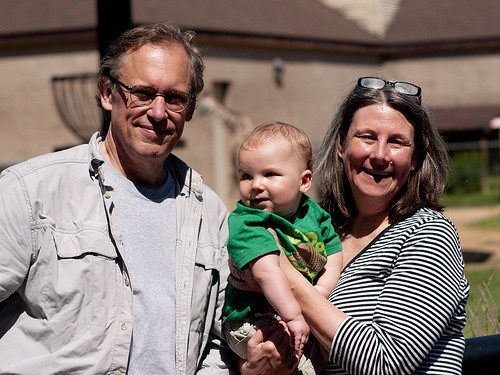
[346,77,421,109]
[107,74,194,112]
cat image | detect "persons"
[220,121,343,375]
[240,76,470,375]
[0,19,230,375]
